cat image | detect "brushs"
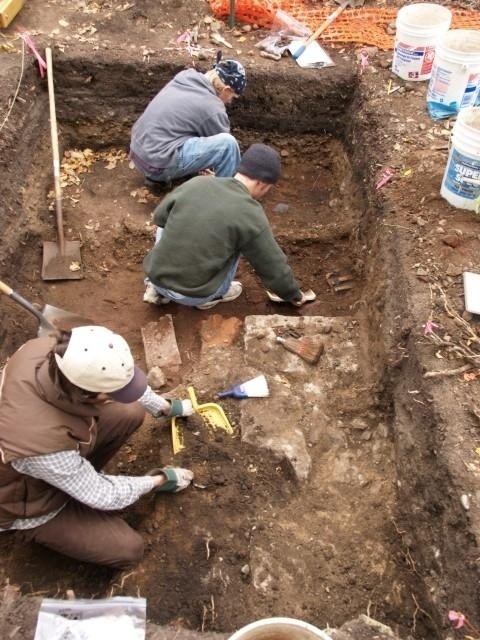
[276,337,324,364]
[217,374,269,399]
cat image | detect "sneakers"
[145,178,173,193]
[143,286,170,305]
[196,281,243,310]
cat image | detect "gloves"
[164,399,193,417]
[147,464,194,494]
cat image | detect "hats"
[237,143,281,183]
[54,326,147,404]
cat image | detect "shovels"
[0,279,95,338]
[40,47,83,280]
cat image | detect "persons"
[129,59,248,194]
[0,325,194,572]
[143,143,306,311]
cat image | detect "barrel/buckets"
[441,106,480,214]
[226,618,337,640]
[427,31,480,118]
[392,3,451,82]
